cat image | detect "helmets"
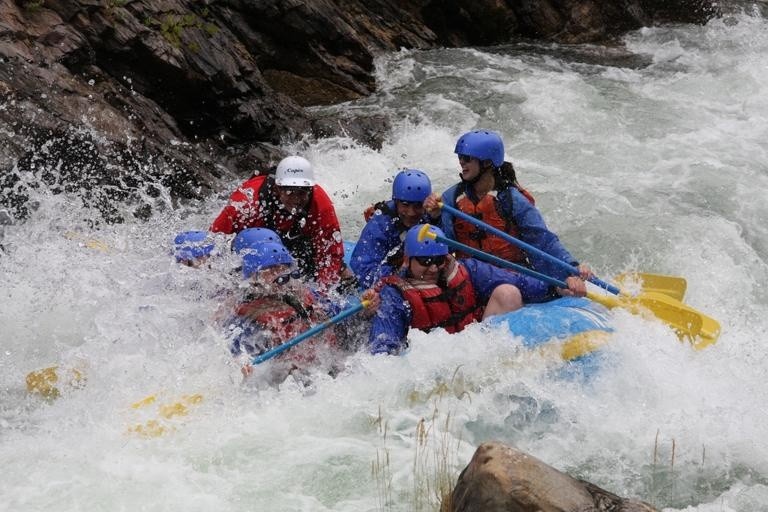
[453,128,505,167]
[405,224,449,257]
[392,168,431,202]
[175,231,219,261]
[231,227,293,277]
[273,155,316,189]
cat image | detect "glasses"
[417,255,446,265]
[457,153,473,162]
[271,270,301,284]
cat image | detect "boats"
[334,241,617,388]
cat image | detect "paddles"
[417,222,702,346]
[607,271,688,306]
[128,298,374,439]
[436,200,722,346]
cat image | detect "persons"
[424,129,598,282]
[232,227,281,253]
[209,156,354,295]
[220,243,381,386]
[174,231,220,269]
[351,169,431,290]
[370,225,586,354]
